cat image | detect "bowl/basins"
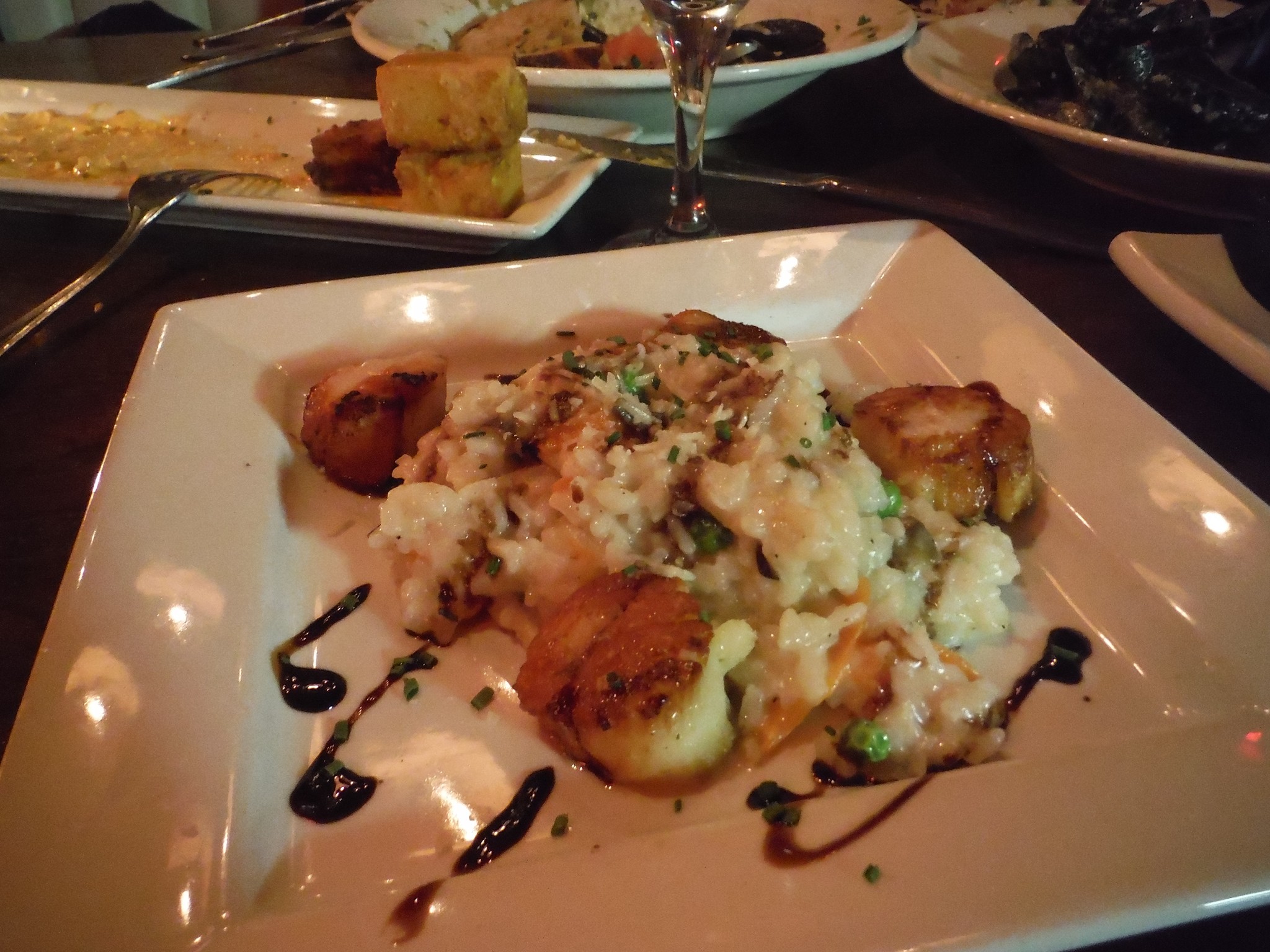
[349,0,918,145]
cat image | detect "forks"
[0,170,281,367]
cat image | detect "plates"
[900,3,1270,179]
[0,72,644,258]
[0,216,1269,950]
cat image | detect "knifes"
[125,26,352,88]
[526,127,1096,254]
